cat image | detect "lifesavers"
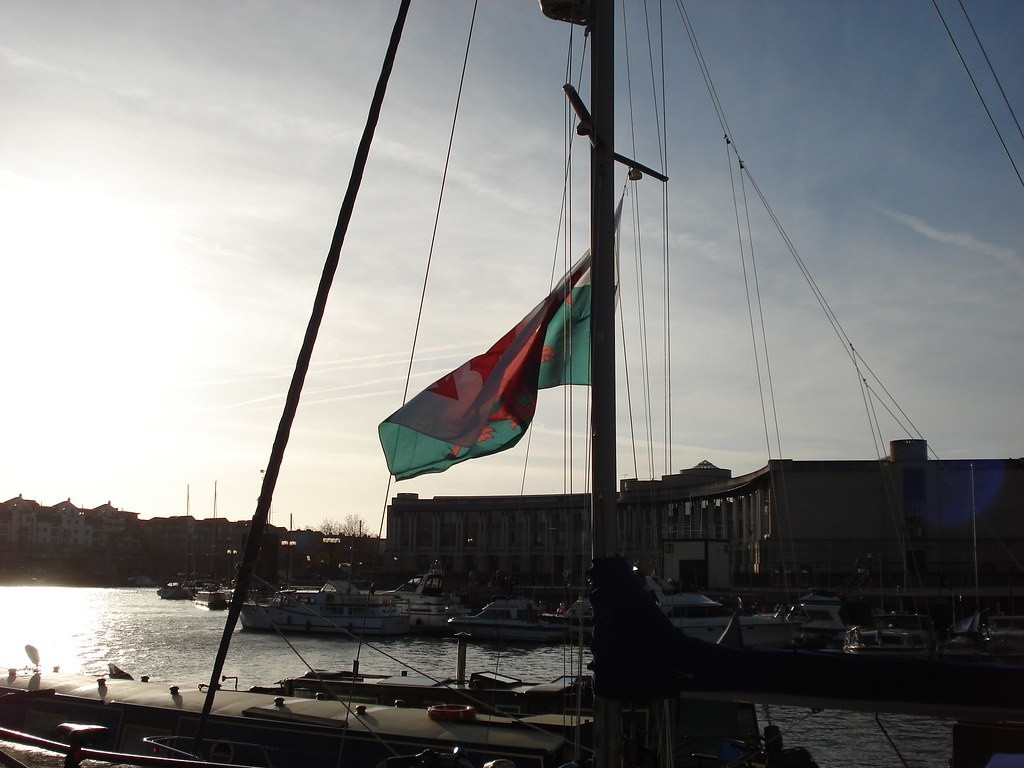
[428,703,477,723]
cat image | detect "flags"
[379,196,625,481]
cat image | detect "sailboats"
[0,0,1024,768]
